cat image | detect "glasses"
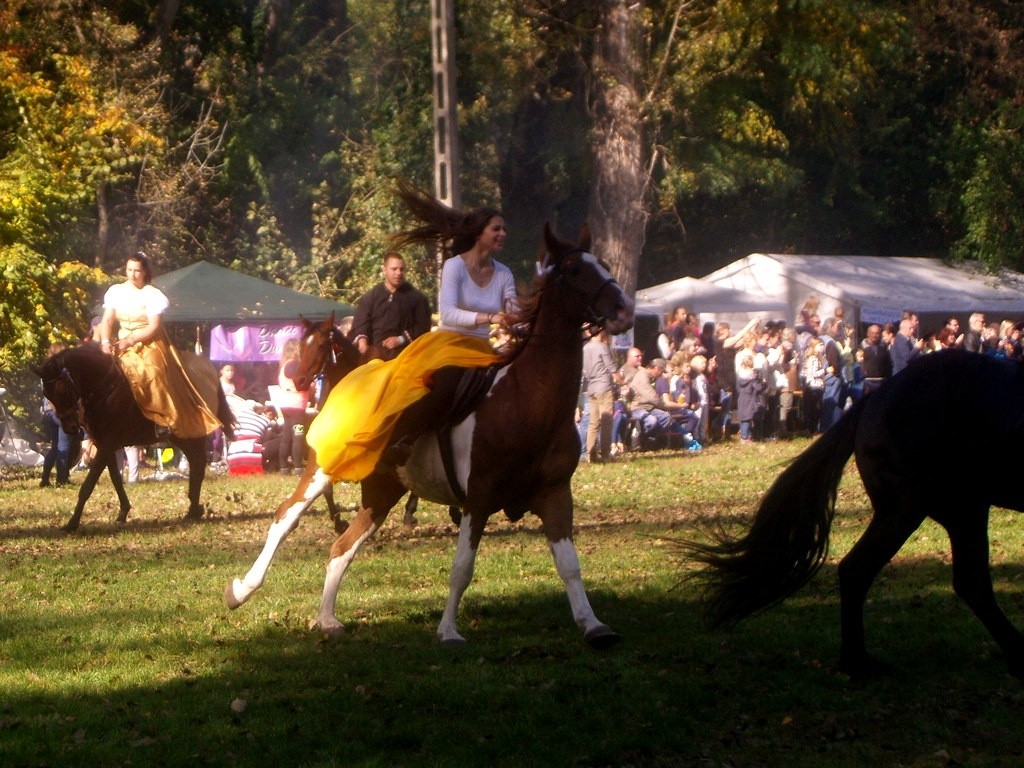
[811,320,821,324]
[977,320,985,323]
[633,354,643,357]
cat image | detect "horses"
[634,345,1024,696]
[27,344,242,535]
[223,217,640,653]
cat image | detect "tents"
[92,261,363,353]
[624,249,1024,329]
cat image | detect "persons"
[198,315,355,475]
[40,317,142,487]
[98,252,223,440]
[348,253,431,372]
[306,186,522,483]
[574,298,1024,461]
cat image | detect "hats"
[651,358,669,374]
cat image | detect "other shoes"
[738,436,756,448]
[281,467,291,476]
[584,449,617,466]
[382,428,418,466]
[685,431,702,453]
[612,444,629,458]
[156,426,169,437]
[293,468,304,476]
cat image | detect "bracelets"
[101,339,110,345]
[488,313,494,323]
